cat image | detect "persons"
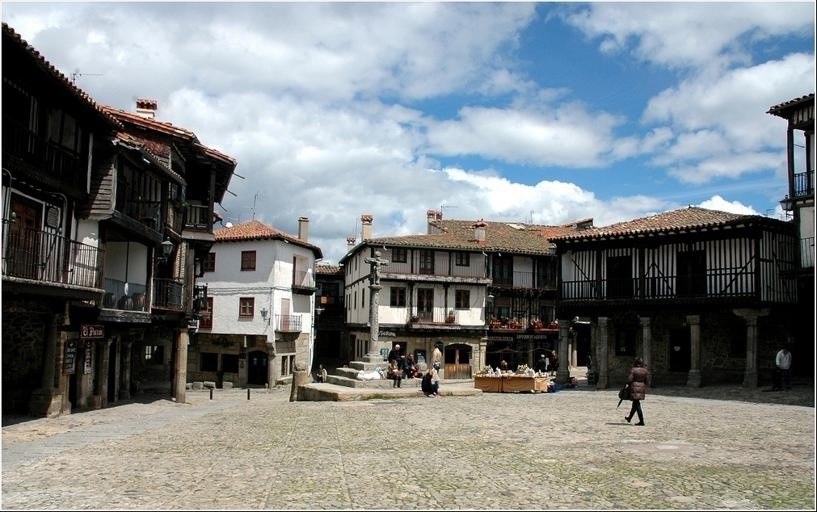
[432,344,443,376]
[624,357,650,426]
[535,354,545,372]
[388,344,422,387]
[316,364,327,383]
[422,369,439,397]
[776,346,793,391]
[550,350,558,376]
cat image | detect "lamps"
[214,216,234,229]
[155,235,173,267]
[259,306,272,327]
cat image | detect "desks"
[474,372,549,394]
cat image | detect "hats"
[634,356,643,366]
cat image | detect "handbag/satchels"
[618,387,631,400]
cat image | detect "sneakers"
[634,421,644,426]
[625,417,632,422]
